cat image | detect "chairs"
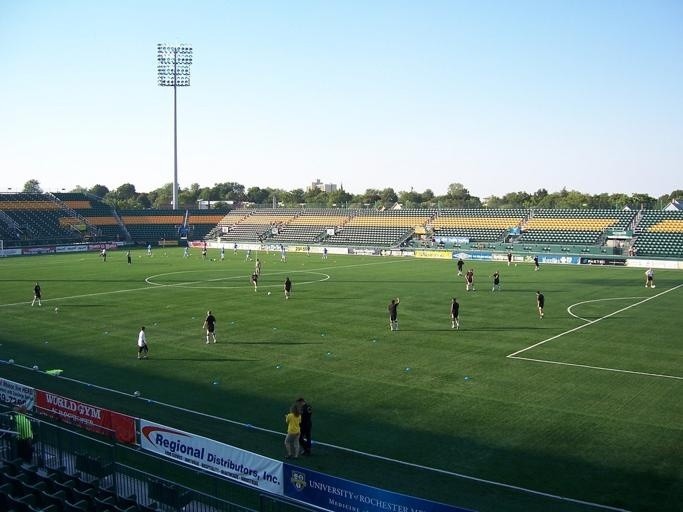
[0,405,196,512]
[0,193,683,258]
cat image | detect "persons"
[490,270,501,293]
[285,404,300,461]
[645,267,656,288]
[94,241,329,300]
[536,291,545,320]
[450,298,460,329]
[465,268,477,292]
[32,283,42,307]
[203,310,218,346]
[296,398,314,456]
[457,258,464,277]
[534,256,540,271]
[507,253,512,266]
[388,297,400,331]
[137,326,149,360]
